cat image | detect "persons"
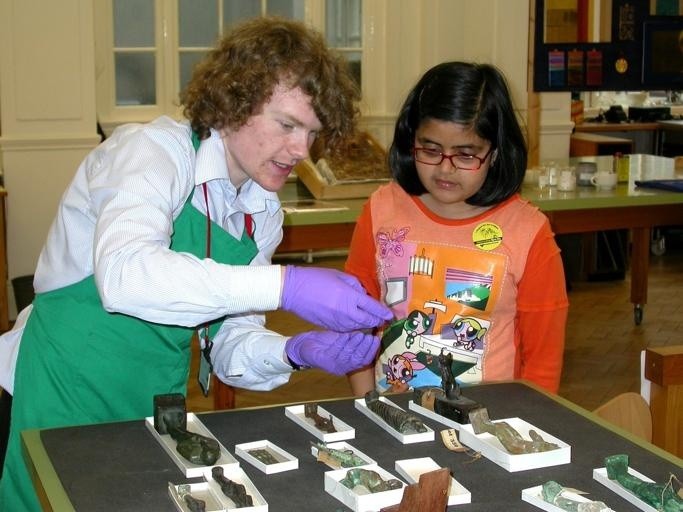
[1,13,395,512]
[345,62,569,392]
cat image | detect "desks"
[14,379,683,511]
[212,154,682,403]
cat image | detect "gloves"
[281,264,393,332]
[285,330,382,377]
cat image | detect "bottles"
[530,161,597,192]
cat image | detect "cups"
[591,171,617,192]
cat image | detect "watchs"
[286,353,311,371]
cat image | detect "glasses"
[412,143,495,171]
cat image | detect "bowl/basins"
[627,91,648,106]
